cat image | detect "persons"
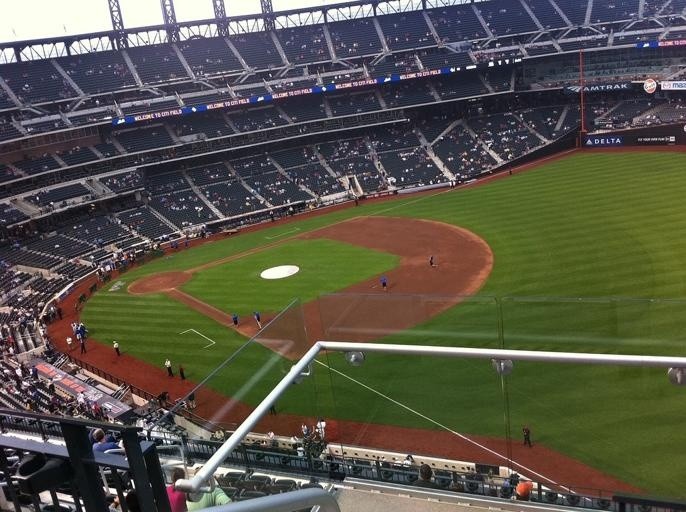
[0,249,140,353]
[0,0,686,253]
[1,350,533,512]
[252,311,260,325]
[380,275,388,292]
[232,313,239,329]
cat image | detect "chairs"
[0,1,686,511]
